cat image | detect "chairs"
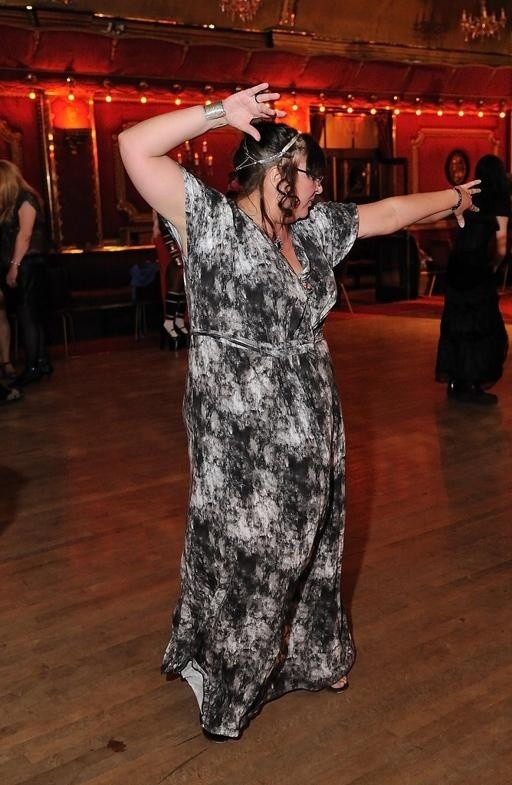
[13,264,78,366]
[135,269,162,342]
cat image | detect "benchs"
[30,251,157,343]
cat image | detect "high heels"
[159,324,190,351]
[8,362,52,388]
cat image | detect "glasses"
[294,167,324,184]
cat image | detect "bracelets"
[451,186,462,210]
[202,100,230,129]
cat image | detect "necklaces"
[258,224,286,253]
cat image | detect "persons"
[0,159,56,405]
[435,156,511,409]
[158,214,191,351]
[118,79,481,745]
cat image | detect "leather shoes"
[447,378,498,404]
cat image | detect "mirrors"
[34,92,104,253]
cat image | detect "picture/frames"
[111,122,157,225]
[0,118,24,176]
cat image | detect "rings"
[254,95,260,104]
